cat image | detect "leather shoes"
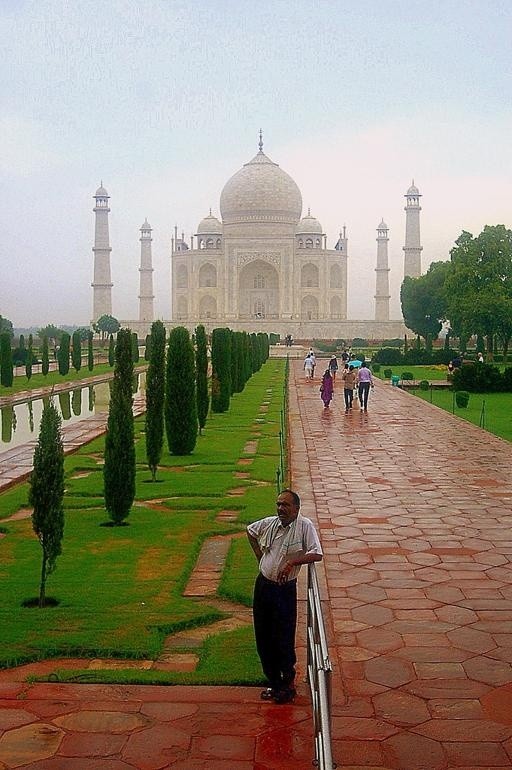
[275,687,296,703]
[260,687,279,700]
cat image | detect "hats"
[330,354,336,360]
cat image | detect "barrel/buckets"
[391,375,400,387]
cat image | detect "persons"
[453,357,461,370]
[448,361,453,374]
[303,345,374,412]
[246,488,322,704]
[476,352,487,365]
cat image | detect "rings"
[281,575,288,578]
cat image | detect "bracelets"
[285,560,294,569]
